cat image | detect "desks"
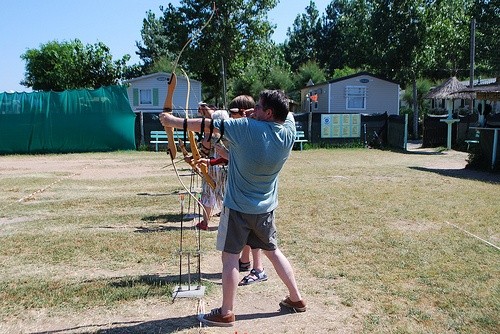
[468,127,500,177]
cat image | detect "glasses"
[255,104,263,110]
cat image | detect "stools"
[465,140,479,153]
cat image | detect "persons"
[161,89,308,328]
[177,94,269,287]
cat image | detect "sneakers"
[197,308,234,327]
[281,296,306,312]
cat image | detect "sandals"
[238,268,268,286]
[239,258,252,272]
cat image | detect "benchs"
[150,130,202,152]
[294,130,309,151]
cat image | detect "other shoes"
[213,212,222,216]
[196,220,208,230]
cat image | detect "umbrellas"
[423,77,477,150]
